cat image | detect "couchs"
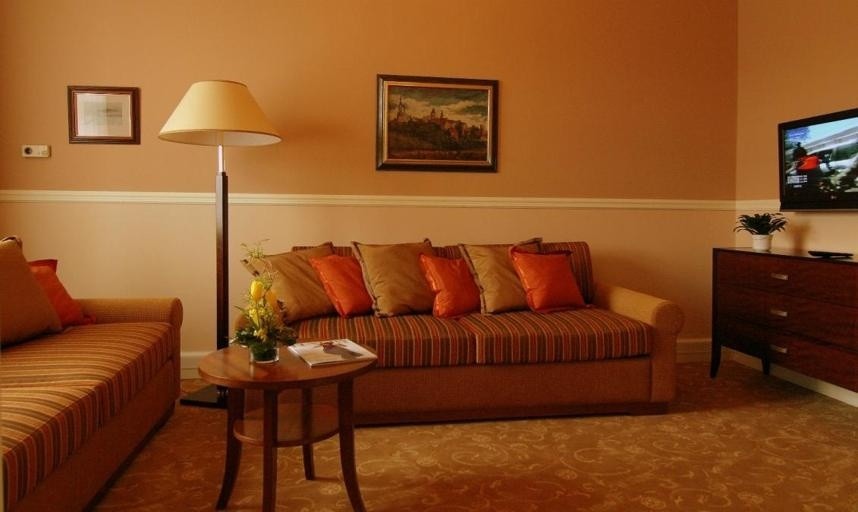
[236,238,686,416]
[0,297,185,512]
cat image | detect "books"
[287,336,378,368]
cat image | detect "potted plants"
[732,211,789,252]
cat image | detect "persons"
[792,141,809,162]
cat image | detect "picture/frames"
[66,83,141,145]
[374,72,500,173]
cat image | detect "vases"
[248,346,282,365]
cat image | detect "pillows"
[238,241,336,327]
[349,237,435,318]
[25,257,95,327]
[504,243,588,315]
[0,233,62,346]
[305,252,372,320]
[415,251,480,319]
[454,237,542,317]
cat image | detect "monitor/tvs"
[777,108,858,213]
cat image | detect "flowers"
[224,240,296,353]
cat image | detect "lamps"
[155,77,283,410]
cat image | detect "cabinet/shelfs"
[709,245,857,392]
